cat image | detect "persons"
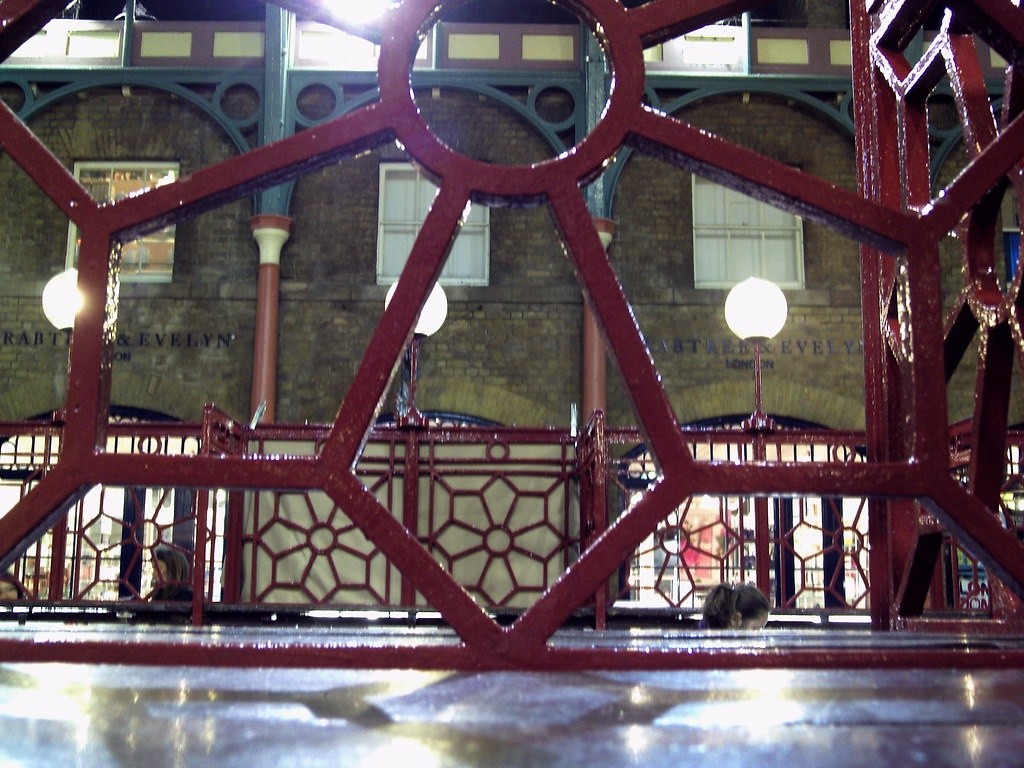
[701,583,769,630]
[149,546,195,601]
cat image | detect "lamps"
[42,266,78,422]
[384,274,448,428]
[725,276,788,430]
